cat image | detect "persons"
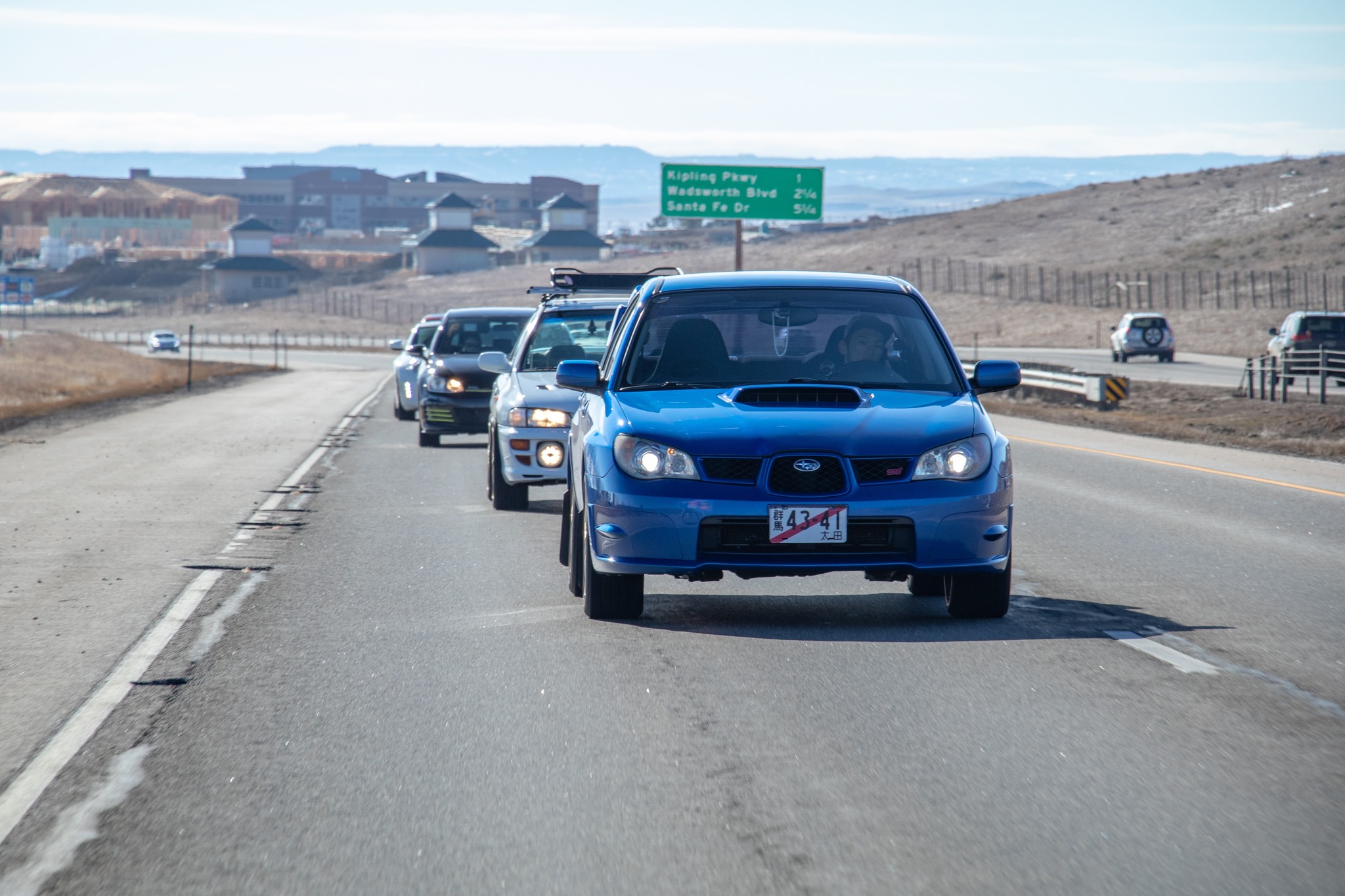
[442,323,481,354]
[810,314,909,386]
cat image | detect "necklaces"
[771,311,791,358]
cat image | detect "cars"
[146,331,181,353]
[389,313,443,420]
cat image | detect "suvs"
[1109,311,1174,365]
[405,306,572,449]
[1266,310,1345,387]
[553,268,1025,624]
[476,265,686,511]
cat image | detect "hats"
[843,314,894,341]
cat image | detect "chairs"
[544,342,588,372]
[638,315,739,388]
[802,322,888,383]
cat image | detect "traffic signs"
[661,161,824,221]
